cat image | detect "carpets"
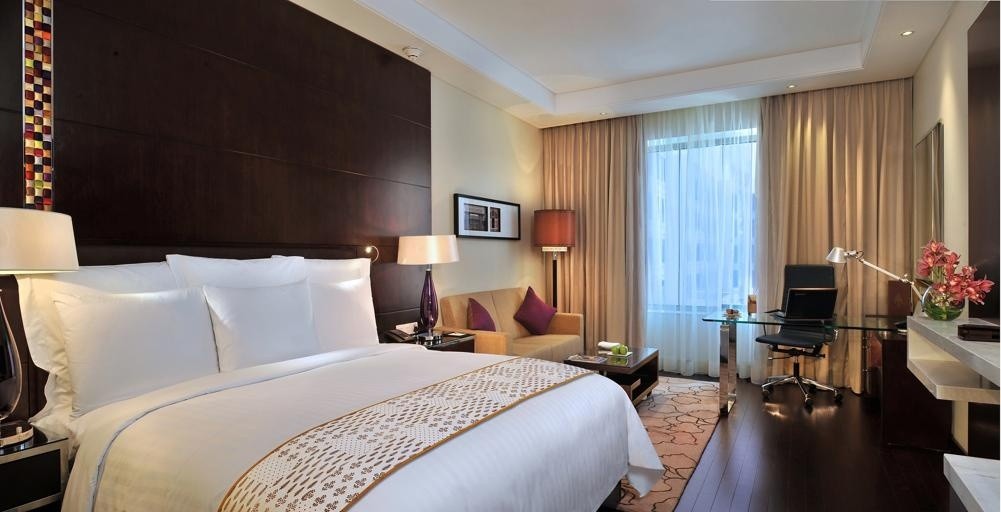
[610,375,735,512]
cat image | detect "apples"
[611,344,628,355]
[610,357,628,364]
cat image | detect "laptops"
[775,288,839,321]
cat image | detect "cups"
[748,294,756,313]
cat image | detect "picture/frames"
[453,191,522,240]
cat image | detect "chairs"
[758,262,845,413]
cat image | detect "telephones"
[383,329,416,344]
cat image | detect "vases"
[920,284,966,322]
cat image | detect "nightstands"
[385,323,476,354]
[0,421,72,512]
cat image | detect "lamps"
[0,207,80,444]
[533,206,576,312]
[826,247,929,312]
[396,230,462,328]
[363,243,380,266]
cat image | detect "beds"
[1,236,661,512]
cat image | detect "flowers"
[914,238,993,309]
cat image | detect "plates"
[720,311,741,316]
[606,350,632,357]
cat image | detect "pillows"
[513,285,556,336]
[270,250,372,283]
[45,283,218,418]
[199,276,320,373]
[311,274,382,358]
[14,261,182,417]
[163,247,308,286]
[465,297,497,333]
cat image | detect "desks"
[903,316,1000,509]
[863,315,927,445]
[702,309,905,416]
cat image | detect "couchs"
[439,285,586,365]
